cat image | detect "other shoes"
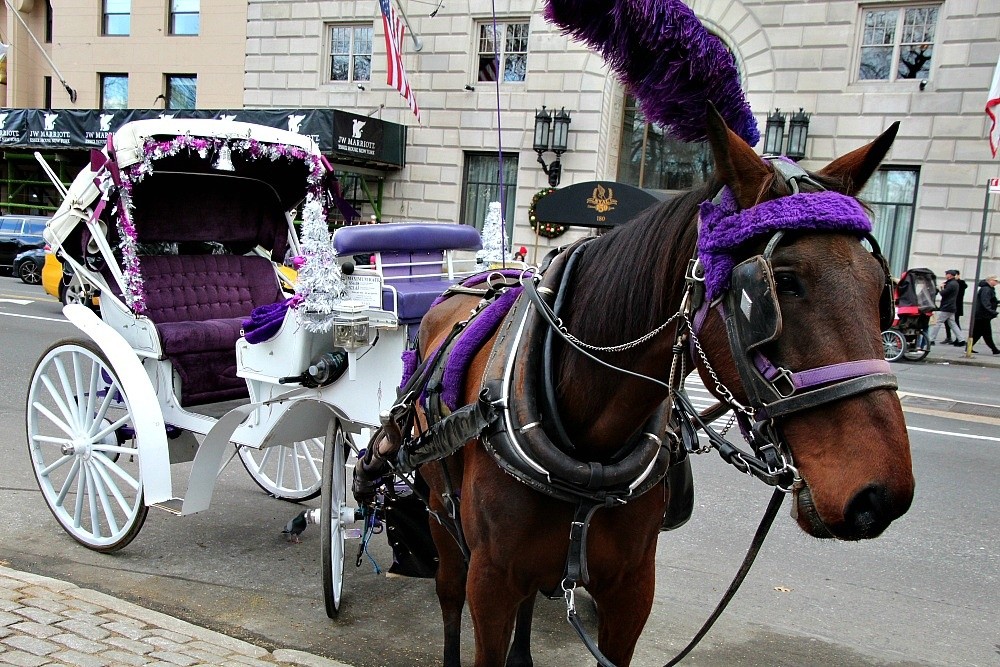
[949,338,958,345]
[940,338,952,344]
[964,349,979,354]
[929,341,935,346]
[954,341,965,346]
[993,349,1000,356]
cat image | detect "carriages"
[22,112,916,667]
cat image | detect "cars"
[12,244,46,285]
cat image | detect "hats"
[945,270,956,276]
[991,274,1000,283]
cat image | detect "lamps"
[533,103,573,184]
[762,106,812,164]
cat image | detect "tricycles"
[880,268,938,363]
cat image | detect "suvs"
[0,214,51,271]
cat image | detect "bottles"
[309,350,346,383]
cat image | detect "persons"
[965,274,1000,354]
[514,246,527,262]
[929,270,965,346]
[940,270,968,345]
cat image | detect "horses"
[414,99,916,667]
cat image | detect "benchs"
[334,220,482,324]
[109,251,285,398]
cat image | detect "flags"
[380,0,420,123]
[986,53,1000,156]
